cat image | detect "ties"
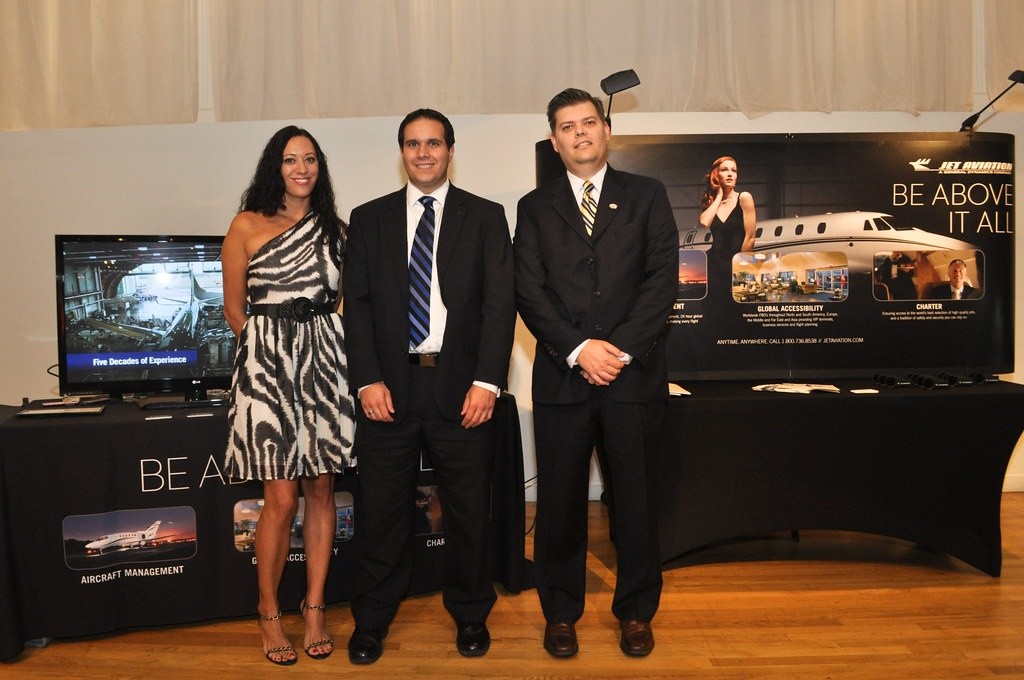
[407,197,437,351]
[576,181,602,236]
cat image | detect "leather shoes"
[618,617,656,658]
[543,620,582,661]
[455,622,492,657]
[348,623,392,666]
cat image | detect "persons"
[698,156,758,369]
[220,125,357,666]
[873,249,986,302]
[340,107,518,664]
[511,86,682,659]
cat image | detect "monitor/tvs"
[54,234,237,409]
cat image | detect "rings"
[367,410,374,415]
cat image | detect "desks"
[639,376,1024,578]
[0,396,529,666]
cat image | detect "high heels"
[255,605,297,668]
[299,596,334,659]
